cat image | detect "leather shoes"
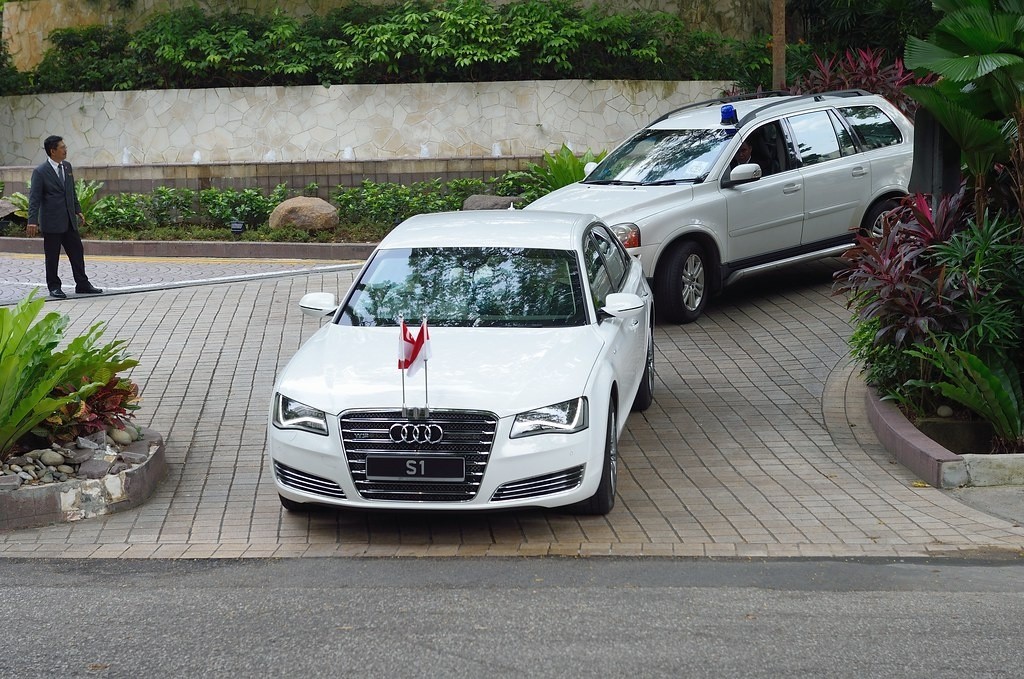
[75,283,102,294]
[50,288,66,298]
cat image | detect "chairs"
[750,132,769,161]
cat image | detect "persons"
[733,139,775,181]
[27,135,104,297]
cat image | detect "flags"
[407,323,431,378]
[398,323,415,370]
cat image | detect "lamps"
[231,221,247,234]
[394,218,403,227]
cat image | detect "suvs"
[521,89,914,325]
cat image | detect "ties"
[57,164,64,188]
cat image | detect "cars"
[267,208,655,517]
